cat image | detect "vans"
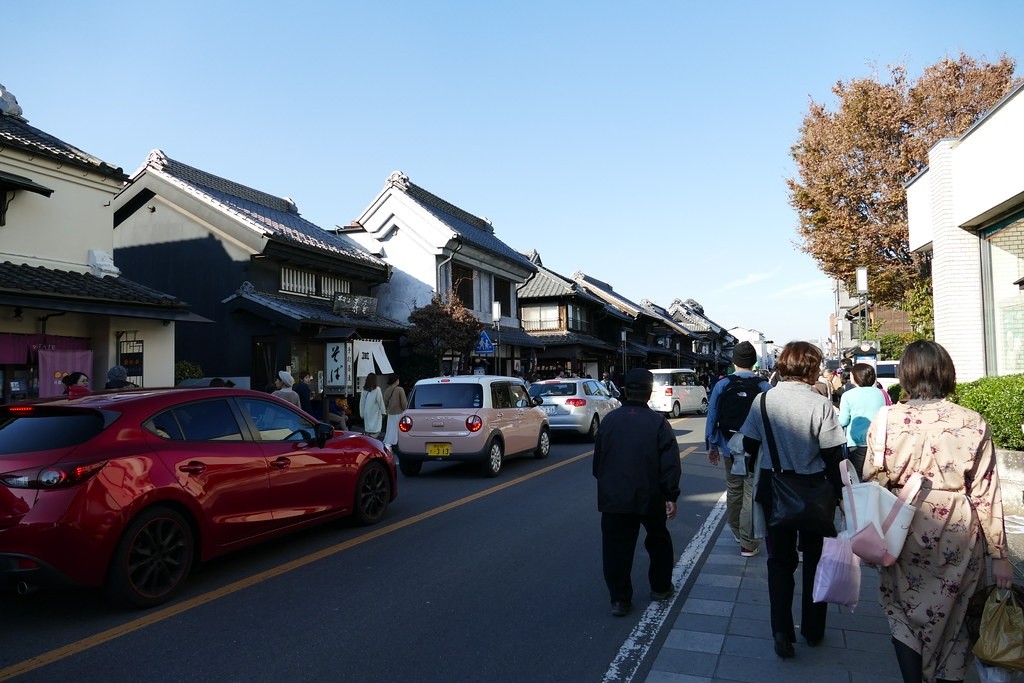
[647,368,709,419]
[876,360,900,392]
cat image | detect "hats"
[733,341,757,367]
[625,368,652,390]
[108,365,127,382]
[279,371,293,386]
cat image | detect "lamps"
[12,307,24,320]
[164,322,170,326]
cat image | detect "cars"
[396,374,552,478]
[0,384,400,614]
[521,377,622,444]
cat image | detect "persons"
[263,371,301,429]
[359,372,385,438]
[600,373,621,398]
[105,366,139,389]
[811,358,891,483]
[863,339,1013,683]
[517,370,531,388]
[759,370,771,383]
[62,372,89,394]
[293,371,313,416]
[593,368,682,615]
[704,341,773,557]
[740,341,848,658]
[555,367,580,378]
[383,374,407,445]
[328,395,348,431]
[696,369,725,388]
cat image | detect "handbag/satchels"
[966,584,1024,683]
[813,532,861,612]
[706,386,710,392]
[839,459,924,568]
[763,471,804,527]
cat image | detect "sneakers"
[741,547,759,557]
[732,531,740,543]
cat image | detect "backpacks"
[713,375,769,441]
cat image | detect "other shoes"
[774,634,795,658]
[651,583,674,598]
[611,598,631,615]
[807,639,818,647]
